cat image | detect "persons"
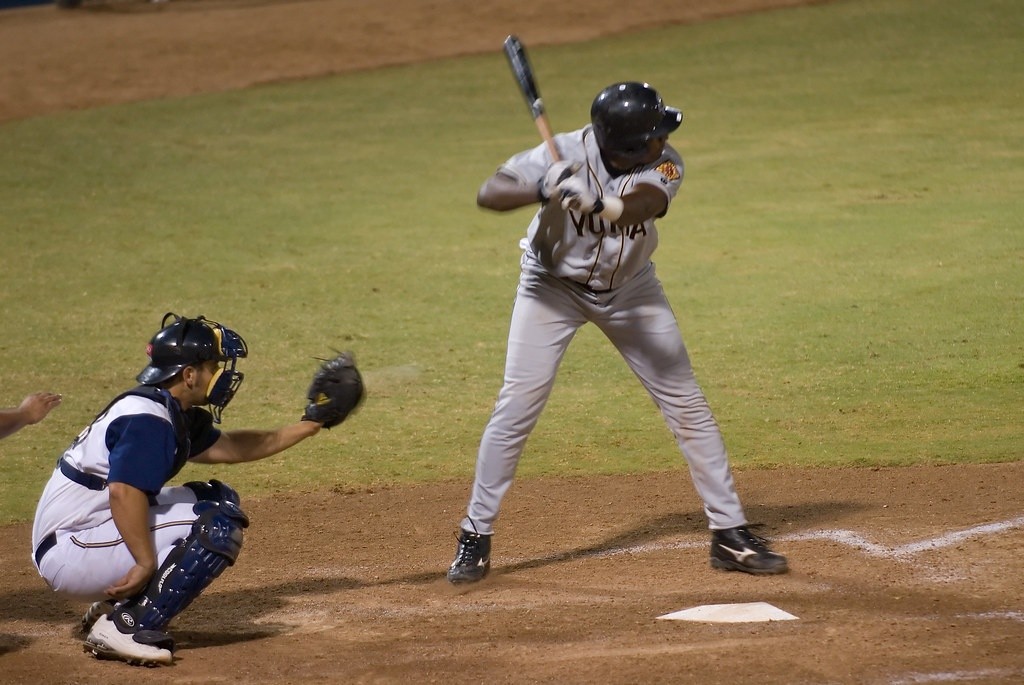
[0,391,63,442]
[30,312,365,668]
[445,81,791,584]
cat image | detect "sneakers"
[709,523,787,575]
[82,613,172,665]
[82,602,115,633]
[447,515,491,583]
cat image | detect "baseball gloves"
[300,342,364,429]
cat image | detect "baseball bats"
[502,34,579,211]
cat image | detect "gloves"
[537,160,583,203]
[556,174,606,216]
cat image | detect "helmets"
[591,82,683,160]
[135,320,219,385]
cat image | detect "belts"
[35,532,56,567]
[564,277,612,295]
